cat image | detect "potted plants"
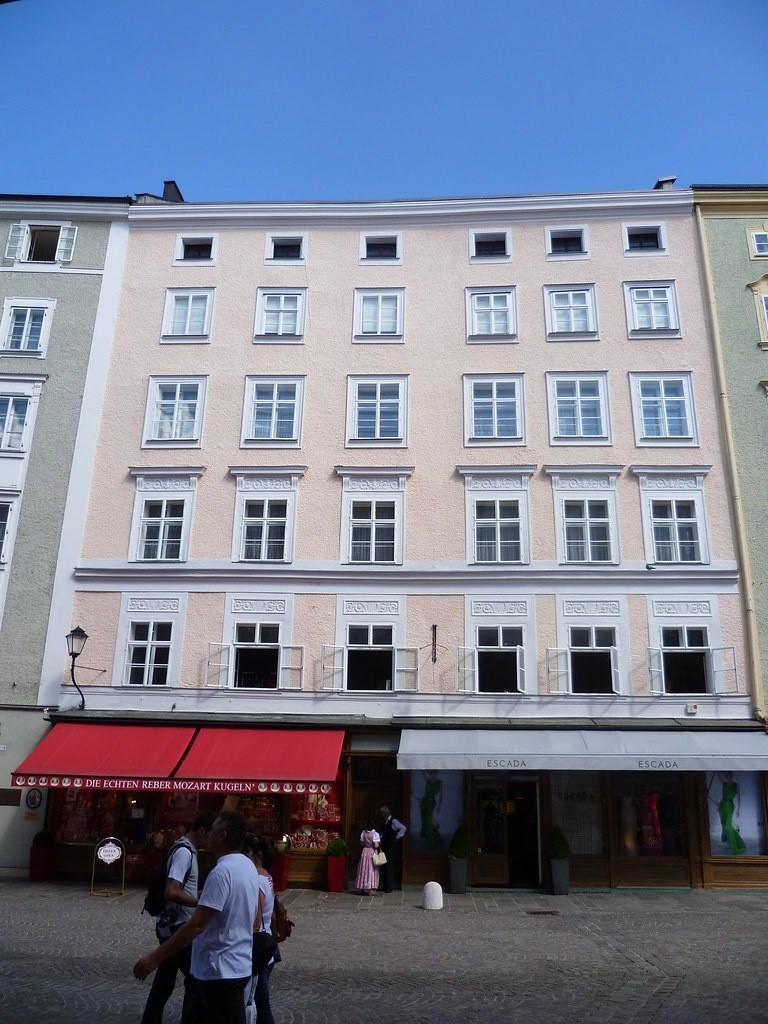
[324,838,349,893]
[29,830,50,882]
[449,824,473,894]
[540,826,571,895]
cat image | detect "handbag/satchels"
[372,848,387,866]
[270,877,294,942]
[252,931,276,977]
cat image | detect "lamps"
[65,626,106,709]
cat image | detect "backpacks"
[142,843,193,915]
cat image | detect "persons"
[132,808,282,1024]
[354,818,382,897]
[377,806,407,893]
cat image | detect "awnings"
[396,726,768,771]
[11,715,346,795]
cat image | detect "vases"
[273,853,290,891]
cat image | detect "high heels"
[369,891,377,897]
[361,890,368,896]
